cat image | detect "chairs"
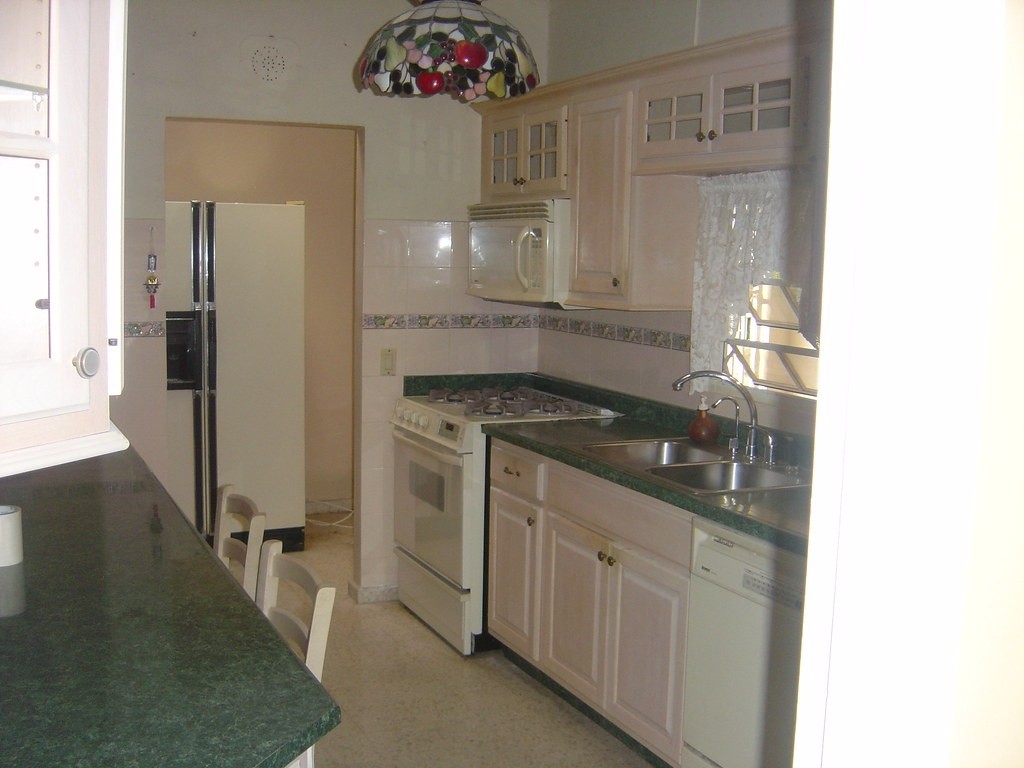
[214,486,337,767]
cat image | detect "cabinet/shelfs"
[470,26,806,312]
[485,436,698,768]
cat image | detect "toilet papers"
[0,505,24,567]
[0,561,26,618]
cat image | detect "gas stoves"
[389,385,626,453]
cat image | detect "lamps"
[358,0,540,105]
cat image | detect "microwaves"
[465,198,596,310]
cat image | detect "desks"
[0,447,341,768]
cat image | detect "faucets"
[672,370,757,459]
[711,396,739,455]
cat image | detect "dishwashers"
[682,517,808,768]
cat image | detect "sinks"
[645,463,811,497]
[558,437,729,468]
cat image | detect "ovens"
[391,428,499,655]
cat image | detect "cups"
[0,505,23,567]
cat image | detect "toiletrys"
[688,395,720,444]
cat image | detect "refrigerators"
[164,198,305,553]
